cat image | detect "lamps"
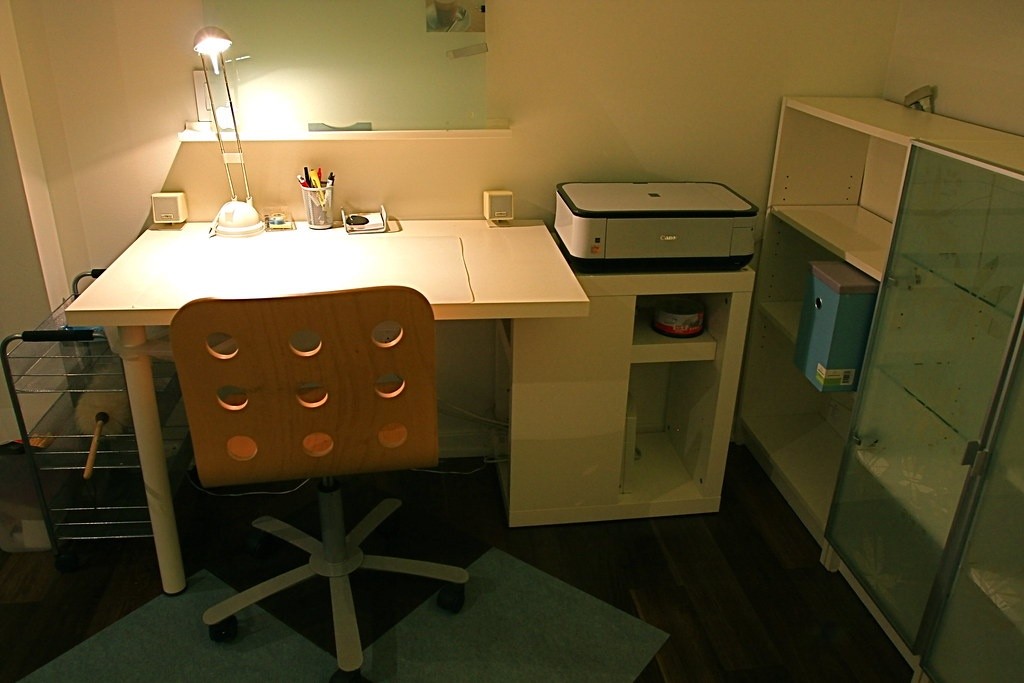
[194,27,267,237]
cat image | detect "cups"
[301,181,333,230]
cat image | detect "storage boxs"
[793,258,878,394]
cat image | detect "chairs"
[169,286,470,682]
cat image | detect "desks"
[65,216,592,596]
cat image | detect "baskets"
[300,180,334,229]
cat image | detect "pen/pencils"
[296,166,335,222]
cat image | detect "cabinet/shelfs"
[727,94,1024,683]
[490,228,756,528]
[1,270,191,570]
